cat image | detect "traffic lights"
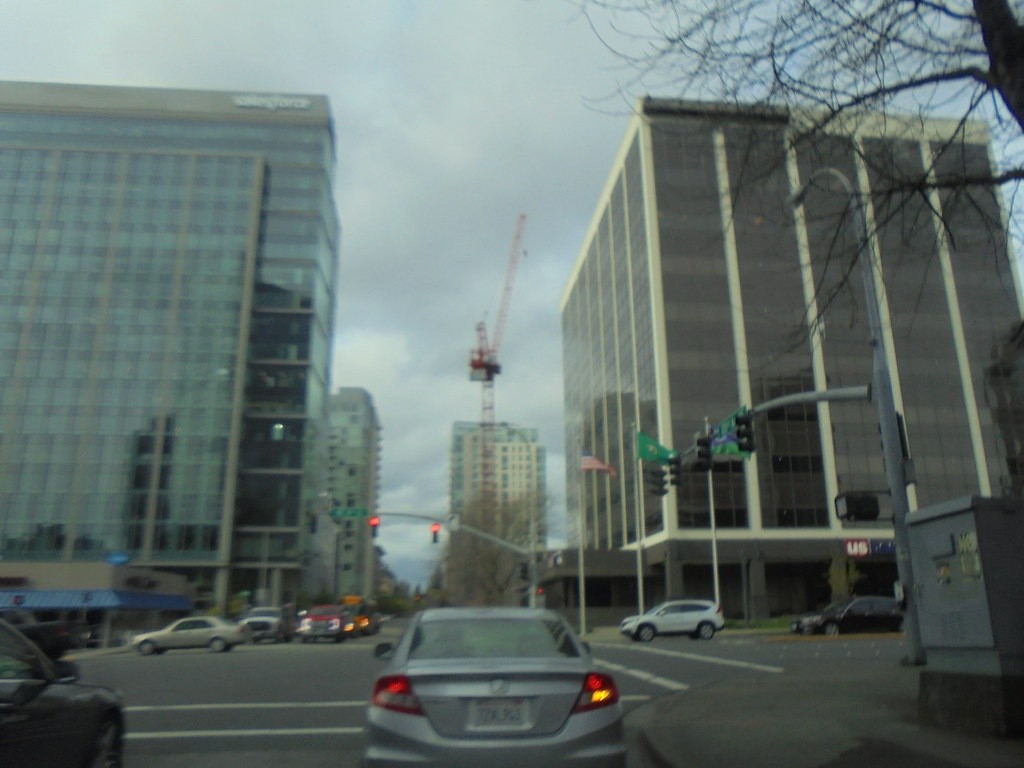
[370,516,378,536]
[695,438,710,472]
[735,414,754,450]
[649,469,668,496]
[431,521,440,542]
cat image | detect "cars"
[130,615,251,655]
[0,613,127,768]
[366,608,628,768]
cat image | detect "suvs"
[621,599,726,642]
[240,609,294,642]
[791,594,903,633]
[296,606,349,642]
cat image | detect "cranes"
[470,216,528,601]
